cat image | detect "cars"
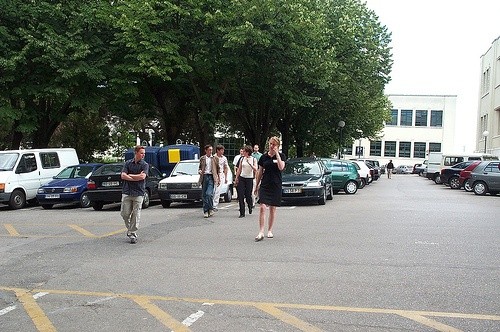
[35,163,103,209]
[380,164,397,174]
[396,165,413,174]
[156,159,234,208]
[87,161,166,211]
[346,156,382,187]
[320,158,361,195]
[280,156,334,205]
[413,151,500,196]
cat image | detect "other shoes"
[255,233,264,241]
[239,213,245,219]
[249,208,253,214]
[203,211,210,217]
[267,232,274,238]
[127,230,138,244]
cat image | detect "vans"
[0,147,81,210]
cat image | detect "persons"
[387,160,394,179]
[121,147,149,244]
[233,145,263,211]
[198,145,228,218]
[235,145,257,218]
[254,137,286,241]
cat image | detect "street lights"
[116,131,122,157]
[337,121,346,159]
[483,131,489,153]
